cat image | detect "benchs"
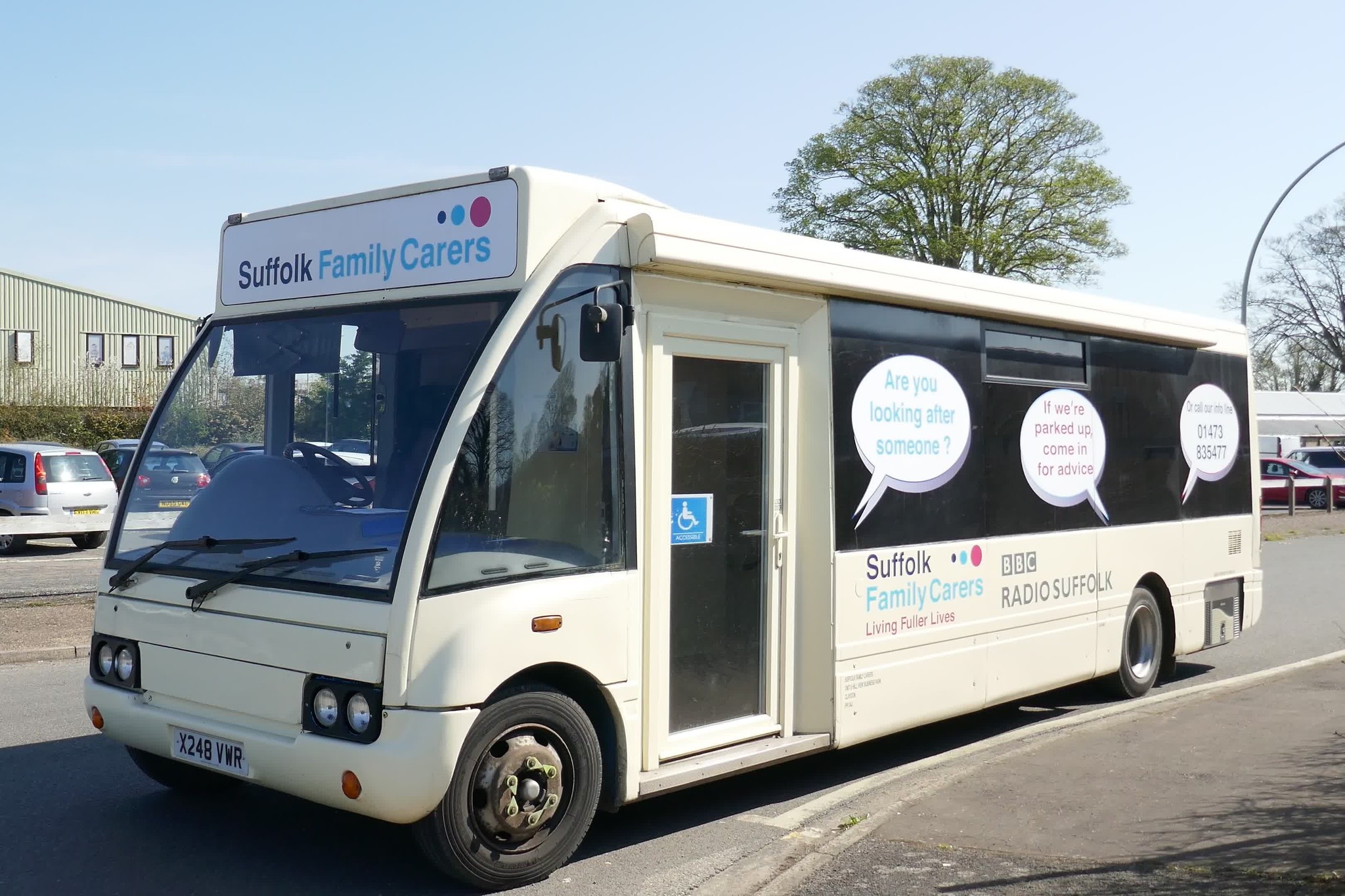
[143,464,187,470]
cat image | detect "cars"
[97,438,380,531]
[1260,457,1345,508]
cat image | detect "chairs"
[382,384,468,531]
[153,466,171,470]
[1272,465,1278,475]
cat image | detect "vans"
[1283,447,1344,483]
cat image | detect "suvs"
[0,440,118,549]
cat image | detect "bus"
[80,166,1261,891]
